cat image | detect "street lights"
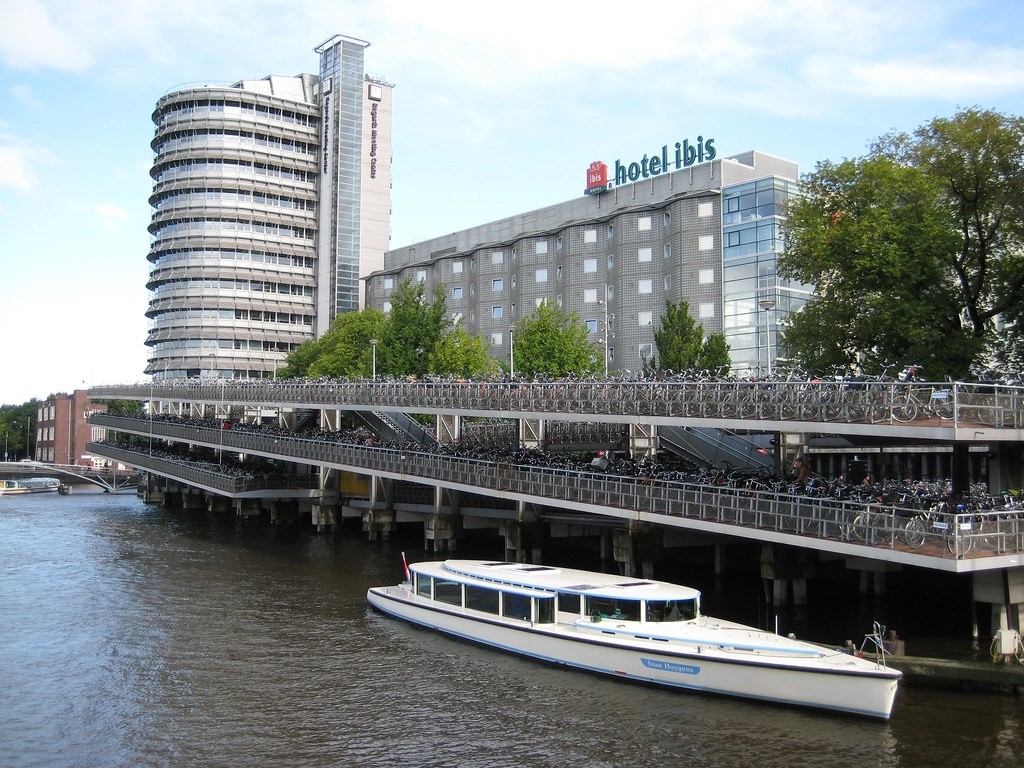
[506,322,518,383]
[270,347,279,380]
[369,338,379,389]
[208,353,215,383]
[596,282,616,381]
[758,299,776,382]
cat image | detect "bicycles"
[95,360,1024,556]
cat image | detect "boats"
[58,483,73,496]
[0,477,61,495]
[365,559,904,724]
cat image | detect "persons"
[104,459,110,474]
[87,461,92,471]
[862,476,870,487]
[794,457,811,493]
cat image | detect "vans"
[20,459,32,462]
